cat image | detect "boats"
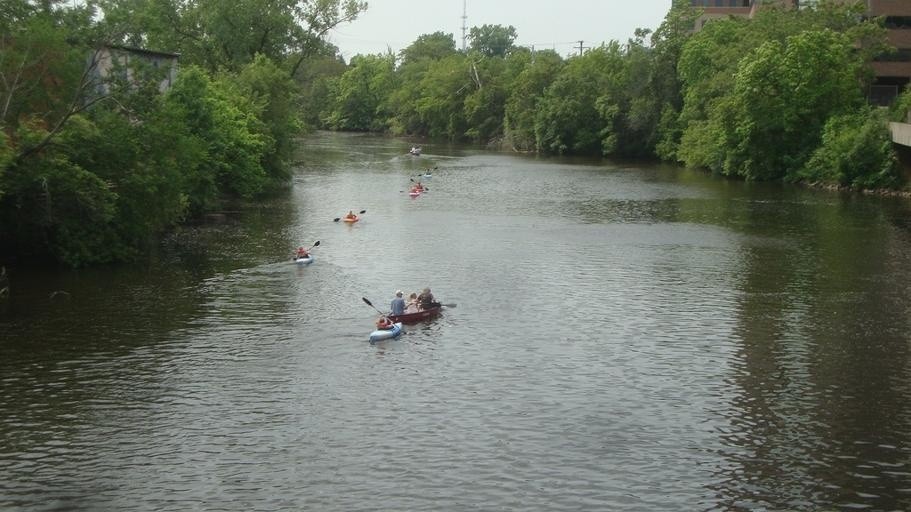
[417,188,424,192]
[368,321,403,342]
[411,148,421,155]
[343,215,360,222]
[409,191,420,196]
[295,253,312,266]
[382,305,442,325]
[424,173,433,179]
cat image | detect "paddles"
[432,302,456,308]
[293,241,318,260]
[334,211,366,222]
[360,296,407,335]
[399,166,438,193]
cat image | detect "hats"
[396,289,404,295]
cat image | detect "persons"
[426,169,431,175]
[296,247,309,258]
[376,315,393,330]
[408,186,417,193]
[346,210,357,219]
[407,293,422,312]
[414,288,441,310]
[391,290,413,314]
[416,182,423,189]
